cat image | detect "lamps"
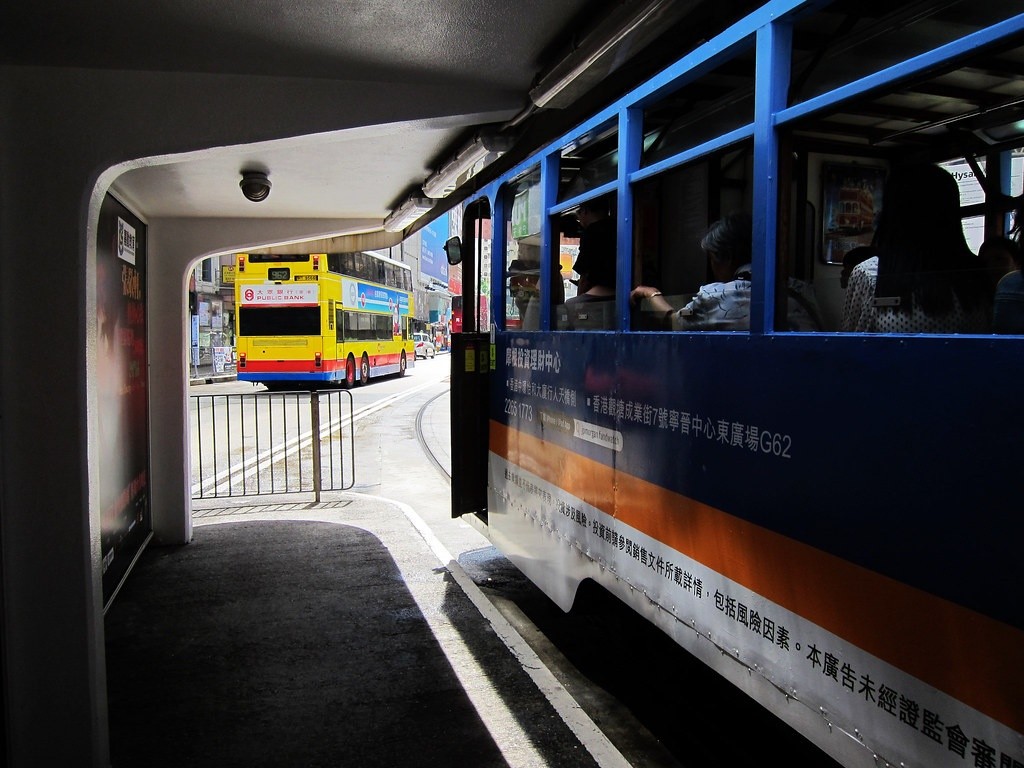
[383,194,437,233]
[529,0,687,110]
[421,132,515,198]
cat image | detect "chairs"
[566,293,689,335]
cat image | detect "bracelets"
[649,292,661,298]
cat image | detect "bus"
[234,246,422,391]
[441,0,1024,768]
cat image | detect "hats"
[573,217,617,277]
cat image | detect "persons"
[514,86,1024,333]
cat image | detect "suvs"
[412,332,436,360]
